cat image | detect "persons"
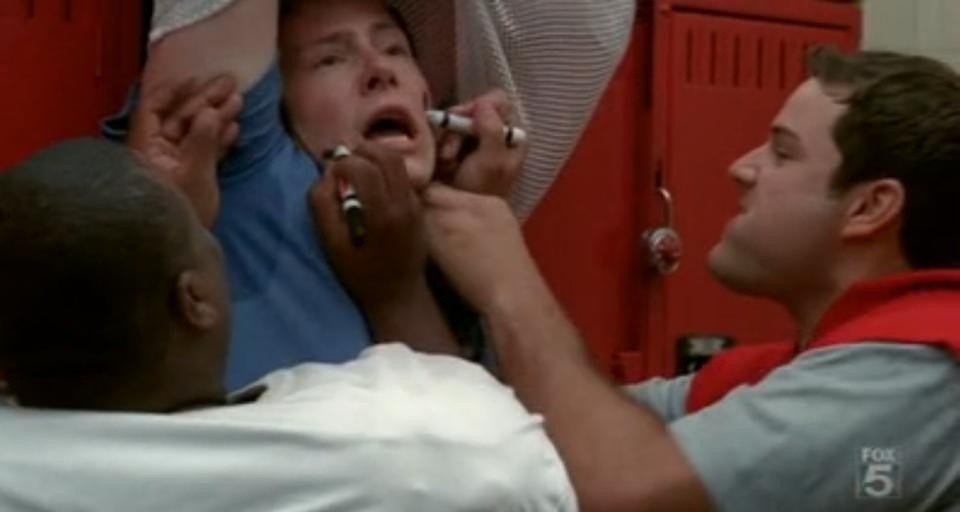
[0,0,579,512]
[425,39,960,512]
[100,0,467,396]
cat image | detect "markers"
[332,145,362,216]
[424,109,527,146]
